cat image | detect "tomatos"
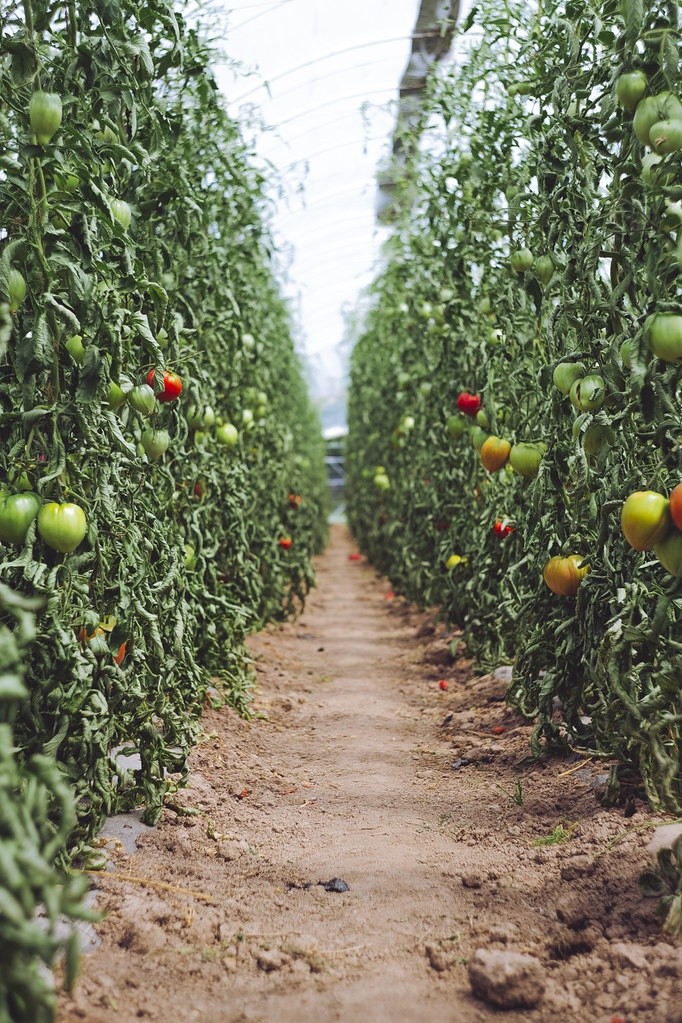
[438,680,449,691]
[376,75,682,595]
[1,93,302,665]
